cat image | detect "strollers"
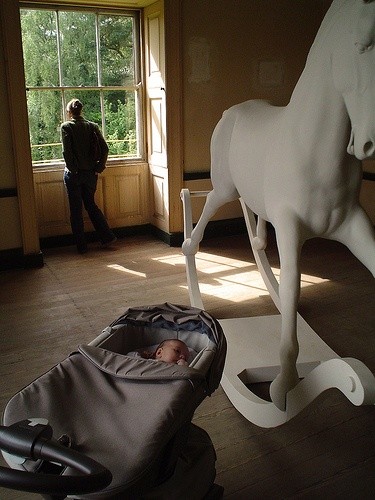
[0,302,225,500]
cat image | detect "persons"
[60,99,116,254]
[147,339,190,367]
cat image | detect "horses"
[180,1,375,413]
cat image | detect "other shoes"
[98,236,118,248]
[77,244,87,254]
